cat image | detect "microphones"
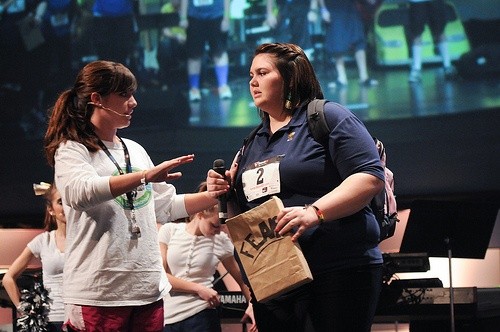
[99,104,131,119]
[213,158,228,225]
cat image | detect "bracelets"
[140,170,149,185]
[308,204,324,225]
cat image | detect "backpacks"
[233,97,400,244]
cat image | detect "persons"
[44,61,239,332]
[3,182,68,332]
[0,0,459,124]
[208,42,388,332]
[158,182,257,332]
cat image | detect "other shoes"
[407,69,422,82]
[218,85,232,99]
[327,80,348,89]
[189,88,202,101]
[359,77,379,87]
[445,67,455,79]
